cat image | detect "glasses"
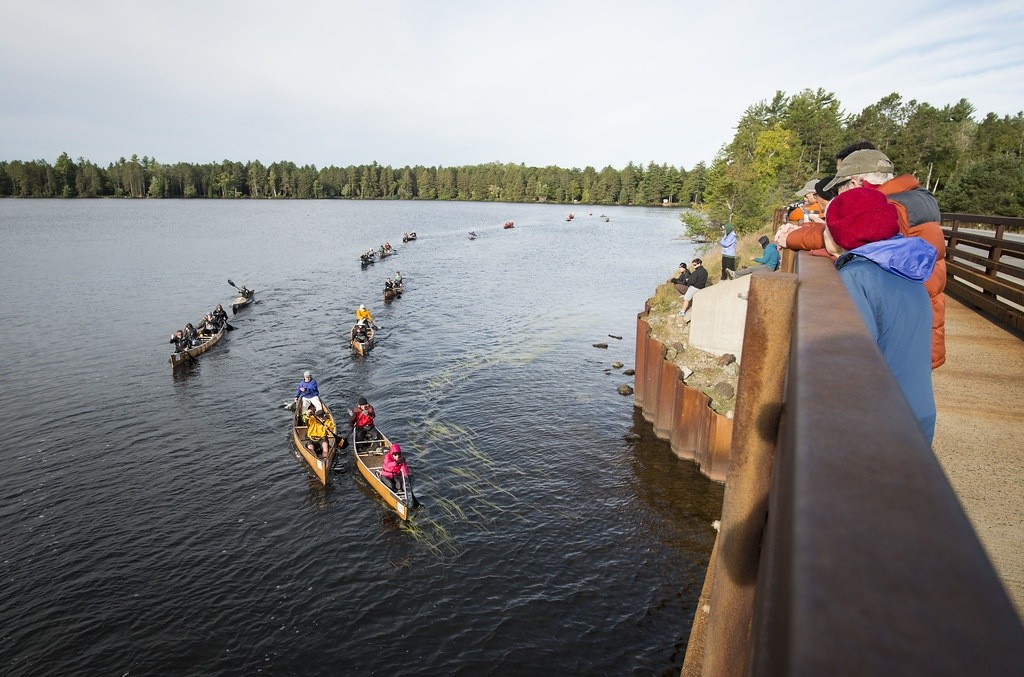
[693,264,698,266]
[813,193,818,199]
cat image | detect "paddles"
[403,460,421,509]
[310,411,350,450]
[173,337,196,364]
[227,279,250,300]
[337,410,365,447]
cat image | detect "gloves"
[405,478,410,483]
[671,278,677,283]
[396,457,405,466]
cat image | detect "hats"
[815,175,839,201]
[826,187,900,250]
[679,262,686,268]
[823,149,894,192]
[304,371,312,376]
[358,397,368,405]
[359,304,366,311]
[316,410,324,417]
[795,179,821,196]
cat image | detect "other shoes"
[358,448,364,452]
[675,311,685,315]
[725,268,736,279]
[372,444,380,451]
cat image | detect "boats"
[384,282,404,301]
[350,318,375,357]
[291,393,338,486]
[362,254,375,268]
[402,235,417,242]
[352,412,414,521]
[379,246,392,258]
[169,311,227,368]
[231,289,254,315]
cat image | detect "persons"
[294,371,323,416]
[385,278,393,291]
[170,331,192,353]
[368,249,375,257]
[468,230,476,235]
[370,322,379,331]
[196,311,220,335]
[238,285,251,299]
[720,222,736,279]
[403,232,407,240]
[671,262,691,285]
[384,242,392,251]
[302,409,334,462]
[378,245,387,254]
[394,271,403,287]
[213,305,229,325]
[775,141,946,447]
[361,253,368,262]
[358,304,372,328]
[410,230,416,237]
[381,442,410,492]
[348,397,375,451]
[184,323,202,345]
[726,235,781,279]
[678,259,708,315]
